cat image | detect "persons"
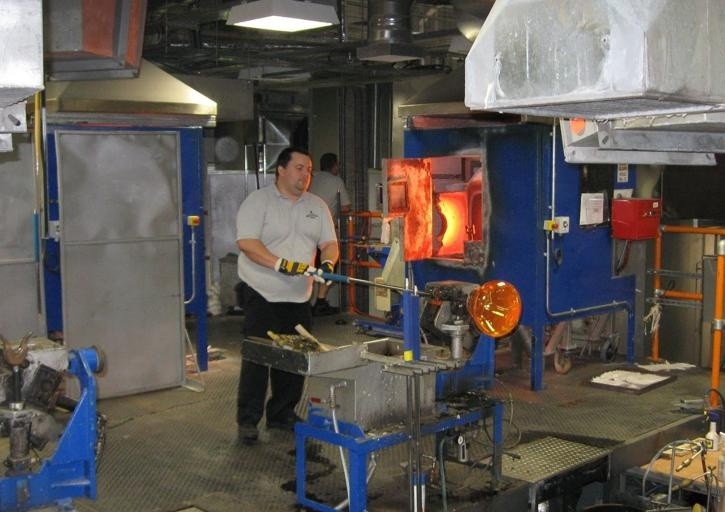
[310,152,353,317]
[233,146,341,442]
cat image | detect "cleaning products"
[703,411,720,452]
[717,431,725,482]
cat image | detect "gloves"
[274,258,334,286]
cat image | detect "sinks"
[341,338,431,363]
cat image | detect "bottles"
[716,448,725,482]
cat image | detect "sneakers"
[237,415,259,442]
[312,297,340,316]
[266,414,305,431]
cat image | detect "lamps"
[225,0,340,32]
